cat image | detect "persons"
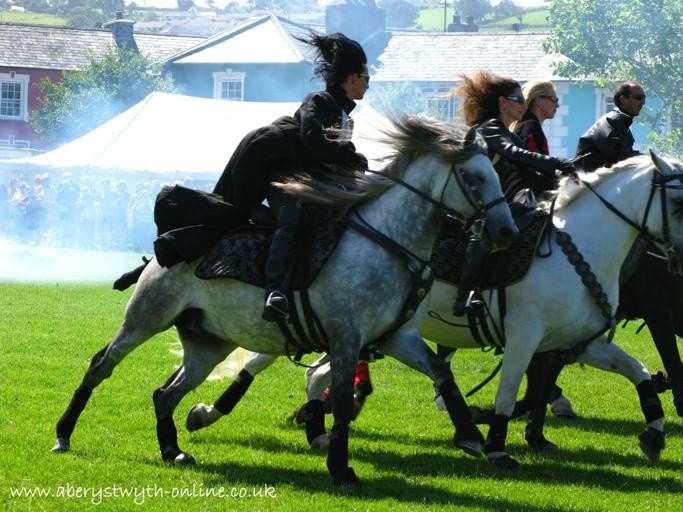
[1,171,197,253]
[513,81,559,156]
[452,78,577,317]
[574,83,646,171]
[240,34,370,321]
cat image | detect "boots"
[262,228,293,322]
[452,238,483,318]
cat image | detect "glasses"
[361,73,371,82]
[625,93,646,101]
[539,94,559,103]
[507,95,525,102]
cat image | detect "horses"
[49,110,519,485]
[185,148,683,465]
[434,225,682,465]
